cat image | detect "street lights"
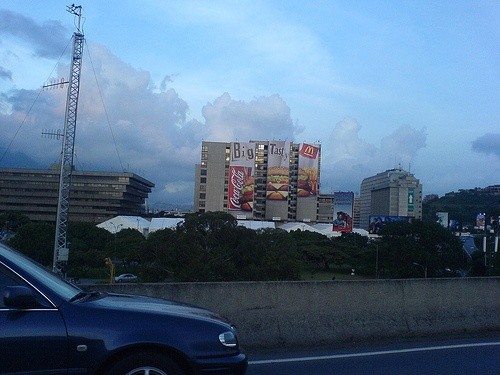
[412,263,427,278]
[367,242,379,275]
[109,221,124,257]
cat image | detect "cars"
[112,273,138,283]
[0,244,247,375]
